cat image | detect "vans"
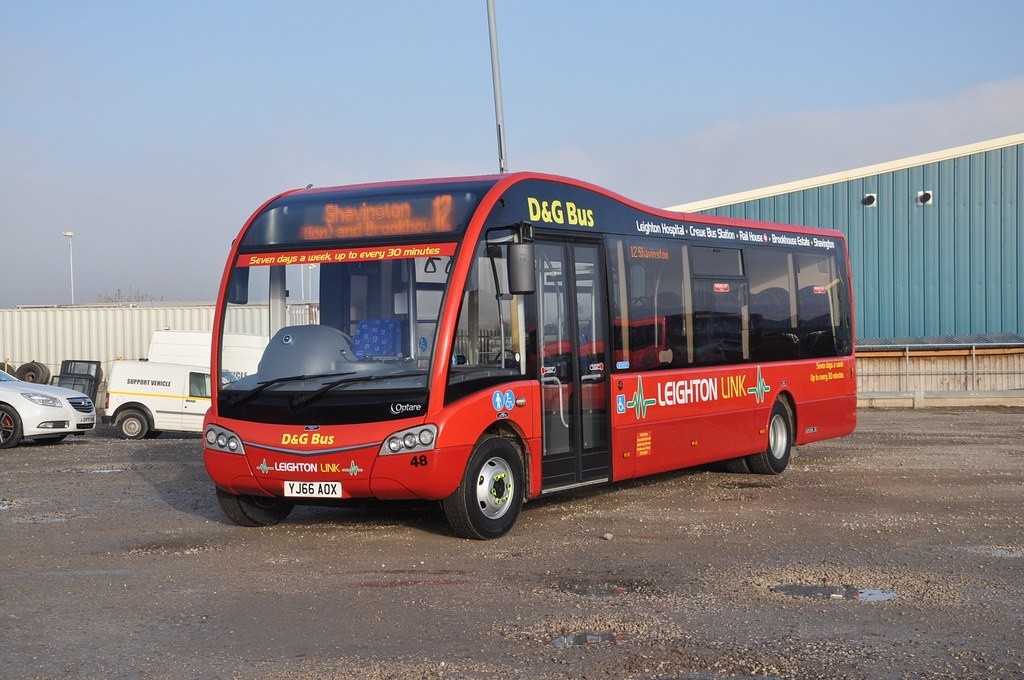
[101,358,240,441]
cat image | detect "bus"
[201,168,855,539]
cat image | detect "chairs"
[348,318,402,360]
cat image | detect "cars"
[0,368,97,449]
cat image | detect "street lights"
[63,231,74,307]
